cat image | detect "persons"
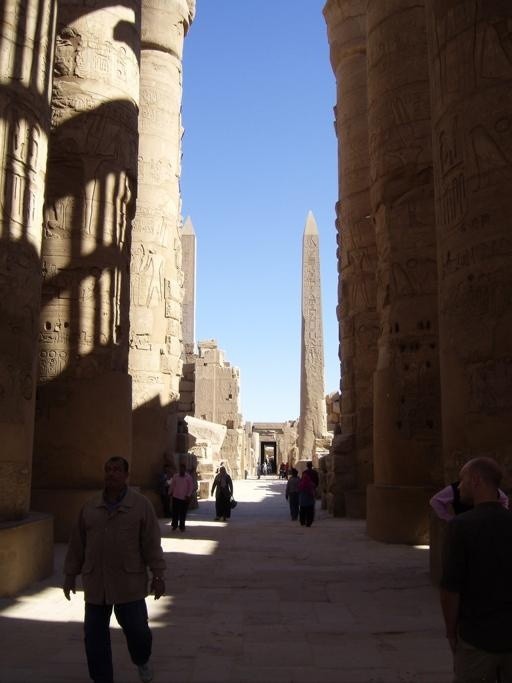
[285,468,299,521]
[256,456,275,480]
[428,477,510,521]
[438,457,512,682]
[297,472,315,527]
[62,456,168,683]
[168,462,194,532]
[300,460,319,488]
[210,466,234,521]
[159,463,173,518]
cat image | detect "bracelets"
[152,575,164,580]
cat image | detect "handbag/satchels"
[230,495,237,508]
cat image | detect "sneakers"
[215,516,227,521]
[300,521,312,527]
[138,663,153,683]
[172,524,185,530]
